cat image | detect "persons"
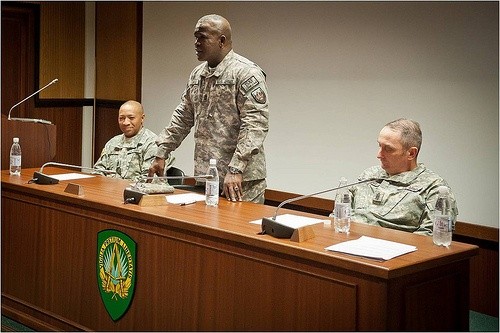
[328,117,458,239]
[91,100,171,185]
[146,14,270,205]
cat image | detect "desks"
[1,167,480,332]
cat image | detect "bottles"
[9,138,21,177]
[432,186,452,247]
[333,179,351,233]
[205,159,219,207]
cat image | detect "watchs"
[228,167,243,174]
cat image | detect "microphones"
[8,79,58,120]
[123,175,213,206]
[33,161,112,185]
[261,178,377,239]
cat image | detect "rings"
[234,186,239,191]
